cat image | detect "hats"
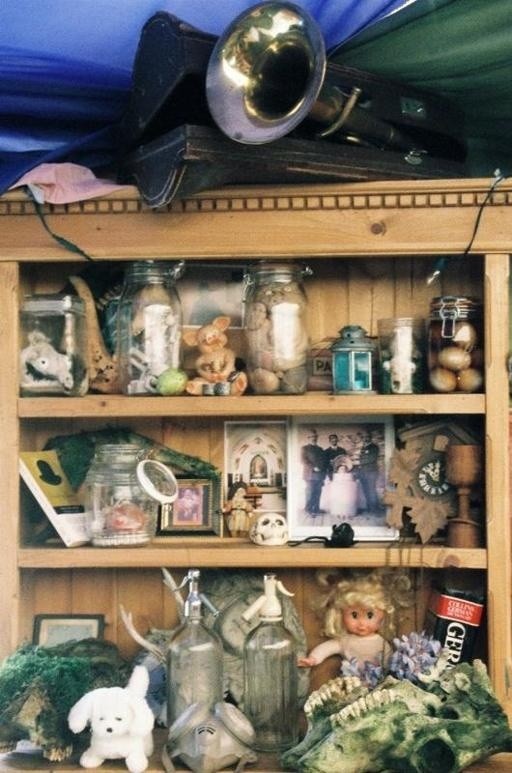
[307,428,318,438]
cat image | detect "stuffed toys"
[67,665,155,773]
[180,313,248,396]
[18,329,75,395]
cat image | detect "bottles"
[242,572,302,751]
[377,317,425,396]
[118,259,183,395]
[425,573,482,674]
[241,257,310,395]
[167,569,225,734]
[17,292,85,398]
[79,443,179,547]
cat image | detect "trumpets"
[206,2,417,156]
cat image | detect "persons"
[220,481,254,538]
[296,568,411,675]
[301,425,383,522]
[177,488,200,519]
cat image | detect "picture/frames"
[27,607,108,648]
[151,472,225,542]
[282,412,402,544]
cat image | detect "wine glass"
[442,444,485,547]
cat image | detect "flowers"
[338,627,442,688]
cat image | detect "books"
[17,450,92,546]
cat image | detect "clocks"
[385,416,484,544]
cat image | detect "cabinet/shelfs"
[0,172,512,772]
